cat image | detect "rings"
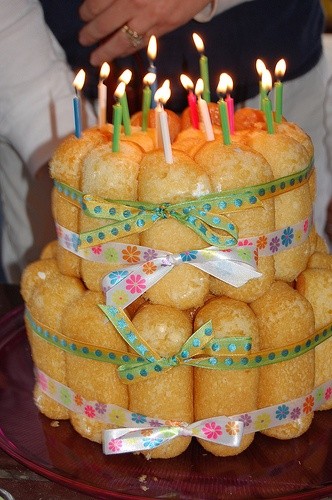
[122,27,143,43]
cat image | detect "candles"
[188,88,200,129]
[200,56,211,102]
[217,97,230,143]
[198,98,214,141]
[98,79,107,127]
[258,79,283,134]
[148,62,158,108]
[155,101,174,162]
[119,90,131,135]
[141,86,151,130]
[226,92,235,136]
[73,87,81,138]
[113,104,122,152]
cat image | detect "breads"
[21,102,332,459]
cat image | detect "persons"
[0,0,332,286]
